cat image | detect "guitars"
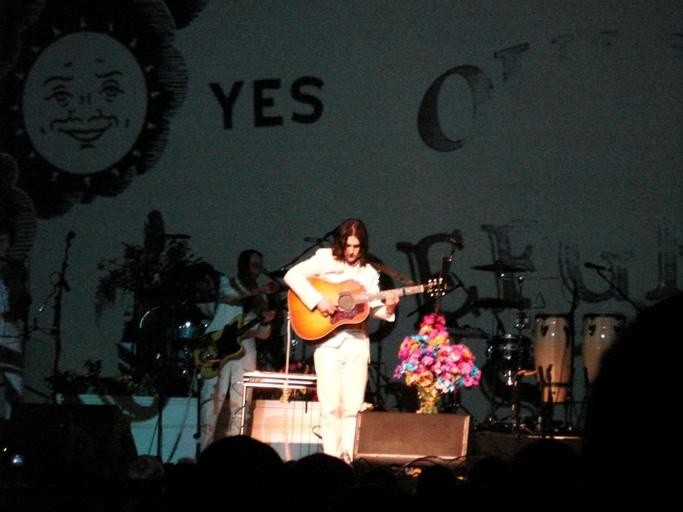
[193,302,284,380]
[288,277,448,340]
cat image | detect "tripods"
[475,297,538,436]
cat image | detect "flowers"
[387,312,482,398]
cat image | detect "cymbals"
[480,296,530,310]
[473,262,533,273]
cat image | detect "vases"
[413,385,445,415]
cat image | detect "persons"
[195,246,279,452]
[0,399,583,512]
[282,217,400,468]
[581,289,681,510]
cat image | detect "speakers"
[352,409,474,465]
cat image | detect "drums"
[480,362,535,407]
[487,334,531,365]
[582,311,626,385]
[534,311,575,405]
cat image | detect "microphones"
[303,235,321,244]
[581,258,607,275]
[260,267,280,283]
[62,228,78,243]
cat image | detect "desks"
[242,393,346,461]
